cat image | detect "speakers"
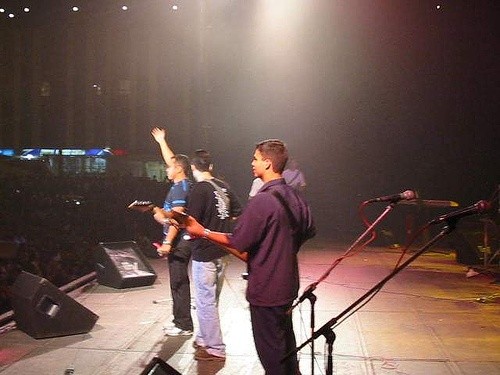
[11,270,99,339]
[94,241,157,289]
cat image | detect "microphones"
[430,200,491,224]
[368,190,414,203]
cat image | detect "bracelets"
[202,229,210,238]
[162,240,172,245]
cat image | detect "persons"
[156,148,243,363]
[151,125,194,337]
[172,138,318,375]
[281,157,307,198]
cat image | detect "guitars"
[128,200,186,231]
[168,210,247,261]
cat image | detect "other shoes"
[163,321,177,329]
[164,327,193,336]
[193,347,226,362]
